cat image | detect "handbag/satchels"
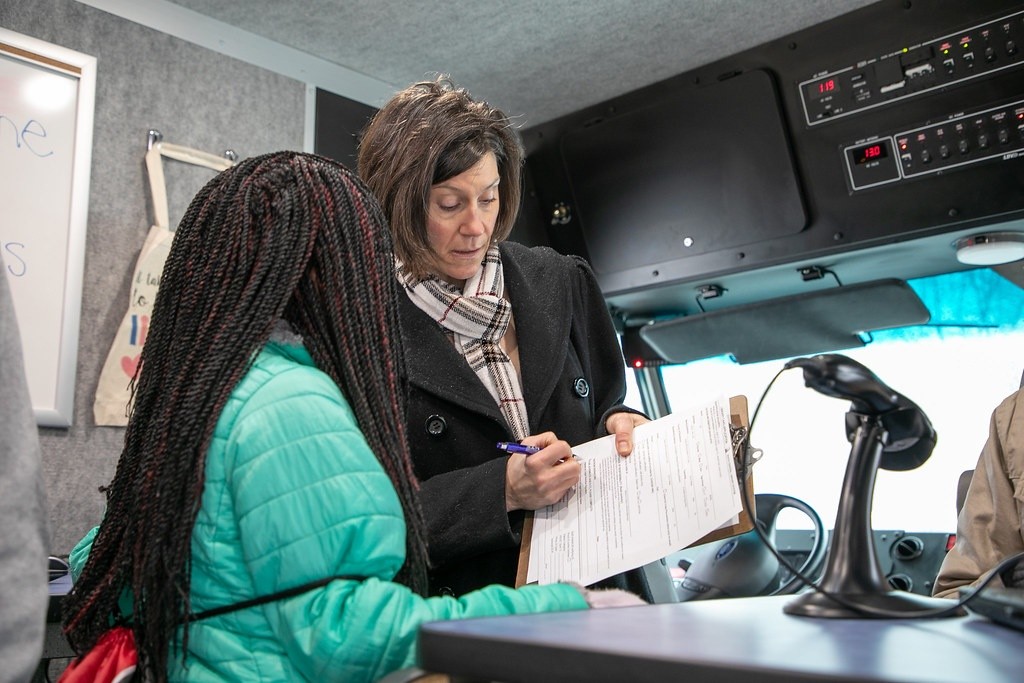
[93,144,239,426]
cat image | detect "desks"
[410,584,1024,682]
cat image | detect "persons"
[63,150,589,681]
[931,384,1023,601]
[352,72,648,600]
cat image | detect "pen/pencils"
[497,441,585,461]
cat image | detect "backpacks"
[60,574,380,683]
